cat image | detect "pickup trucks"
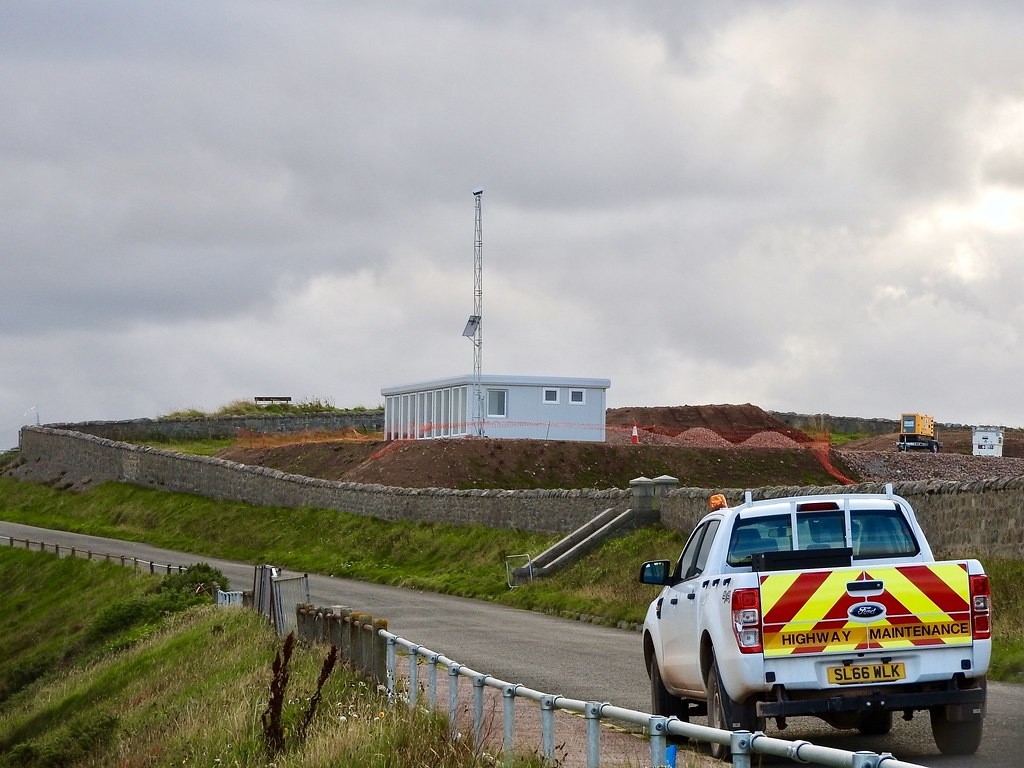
[637,481,993,765]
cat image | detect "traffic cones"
[626,420,640,443]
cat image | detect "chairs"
[731,539,777,558]
[738,529,761,540]
[859,541,889,555]
